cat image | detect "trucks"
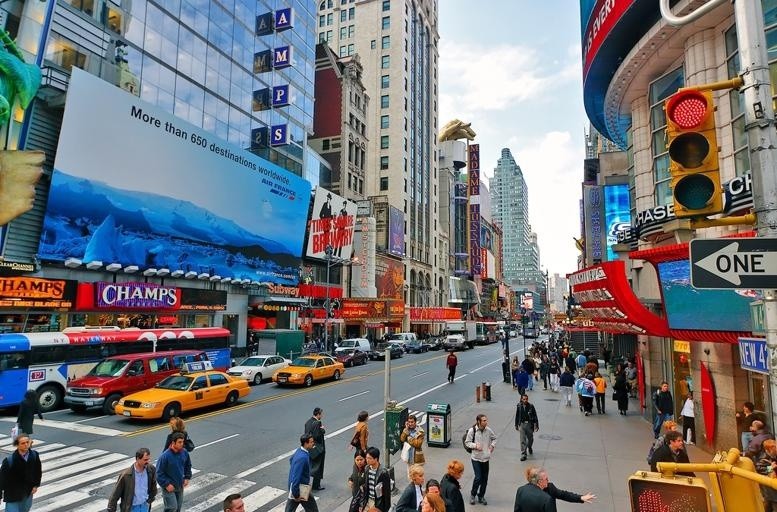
[474,322,499,344]
[443,321,476,350]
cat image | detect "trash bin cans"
[426,402,452,448]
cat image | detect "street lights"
[503,323,511,383]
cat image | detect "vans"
[63,351,212,416]
[334,338,370,356]
[389,333,417,351]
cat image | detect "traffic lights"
[627,471,711,512]
[664,90,724,219]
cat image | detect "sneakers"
[620,411,627,415]
[580,407,604,416]
[469,495,487,506]
[520,448,533,461]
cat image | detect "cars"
[273,355,343,388]
[116,359,250,420]
[305,323,544,369]
[225,354,291,386]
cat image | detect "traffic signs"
[690,236,777,290]
[748,299,767,338]
[738,338,771,376]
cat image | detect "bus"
[0,326,230,408]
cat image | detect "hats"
[428,493,446,512]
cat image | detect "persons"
[0,433,42,511]
[154,433,192,512]
[515,395,539,461]
[305,407,326,489]
[17,390,44,446]
[223,495,245,511]
[396,464,425,512]
[650,431,696,477]
[646,420,677,466]
[446,351,457,383]
[334,201,347,256]
[735,401,777,511]
[319,193,334,254]
[283,434,318,512]
[465,415,497,504]
[420,493,445,512]
[514,468,556,512]
[108,448,157,512]
[679,391,696,445]
[652,381,674,440]
[162,417,187,454]
[364,447,391,512]
[347,410,370,465]
[440,460,465,511]
[400,416,425,482]
[418,479,440,512]
[502,330,638,416]
[348,449,368,512]
[517,466,596,504]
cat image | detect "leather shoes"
[314,487,324,491]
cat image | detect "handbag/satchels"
[350,430,368,449]
[462,427,475,453]
[612,392,617,400]
[185,438,195,452]
[647,443,656,465]
[653,415,664,431]
[401,441,410,463]
